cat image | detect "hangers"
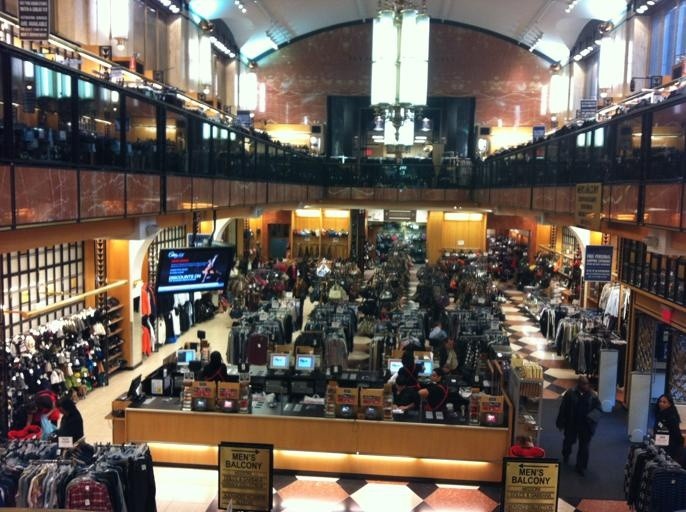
[1,440,147,482]
[635,430,686,473]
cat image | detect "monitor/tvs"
[270,353,289,375]
[127,375,141,402]
[295,354,315,376]
[175,349,195,373]
[414,360,432,383]
[387,359,405,376]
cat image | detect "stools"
[347,350,369,368]
[352,336,373,352]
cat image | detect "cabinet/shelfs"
[515,360,543,459]
[105,303,126,375]
[539,242,582,297]
[291,212,349,261]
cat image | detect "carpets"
[538,396,645,501]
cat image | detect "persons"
[202,350,226,382]
[26,390,60,432]
[202,254,222,282]
[230,259,241,278]
[52,395,83,444]
[393,320,465,410]
[555,374,602,475]
[653,392,686,466]
[274,257,289,273]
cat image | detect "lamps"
[371,0,431,153]
[629,77,662,93]
[198,29,212,95]
[110,0,132,53]
[548,68,562,121]
[597,31,615,101]
